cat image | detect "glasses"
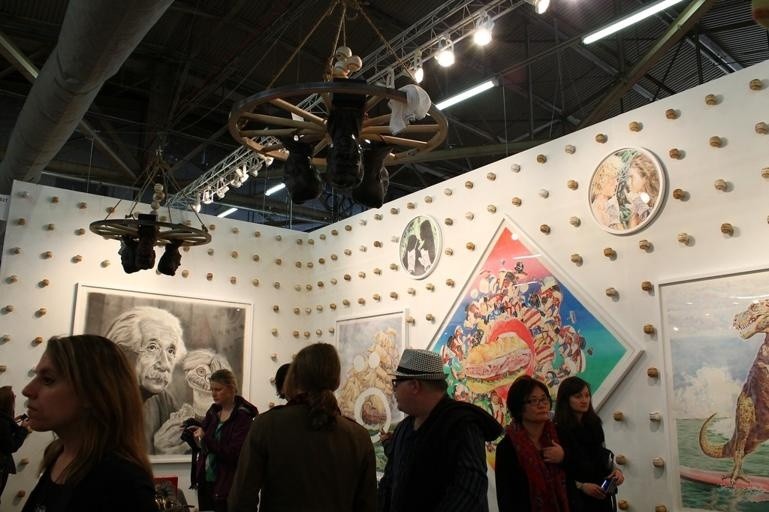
[525,398,551,407]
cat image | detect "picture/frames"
[331,305,409,489]
[654,264,769,512]
[398,214,443,280]
[586,145,666,236]
[422,214,645,489]
[72,281,254,465]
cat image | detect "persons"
[192,368,261,512]
[101,304,187,456]
[439,258,587,454]
[274,363,291,400]
[21,331,162,511]
[552,376,625,512]
[0,385,33,512]
[378,424,393,457]
[374,347,504,511]
[226,342,378,511]
[494,373,578,511]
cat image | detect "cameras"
[600,475,617,495]
[20,413,29,422]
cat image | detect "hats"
[388,349,449,380]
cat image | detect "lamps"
[89,143,211,276]
[404,8,495,83]
[188,142,275,214]
